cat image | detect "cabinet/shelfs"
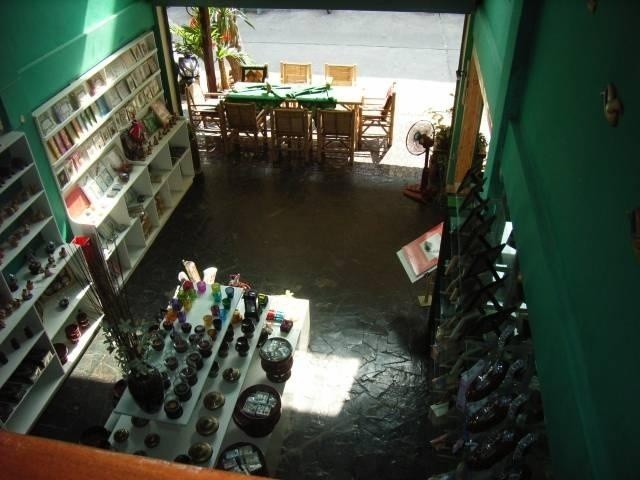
[103,282,311,477]
[32,29,196,304]
[0,130,104,436]
[422,192,555,479]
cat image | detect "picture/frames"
[445,160,516,340]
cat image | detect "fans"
[403,120,438,207]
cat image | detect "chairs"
[185,63,396,170]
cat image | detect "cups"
[162,278,237,353]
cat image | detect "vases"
[128,363,165,414]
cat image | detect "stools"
[110,374,127,408]
[73,423,110,449]
